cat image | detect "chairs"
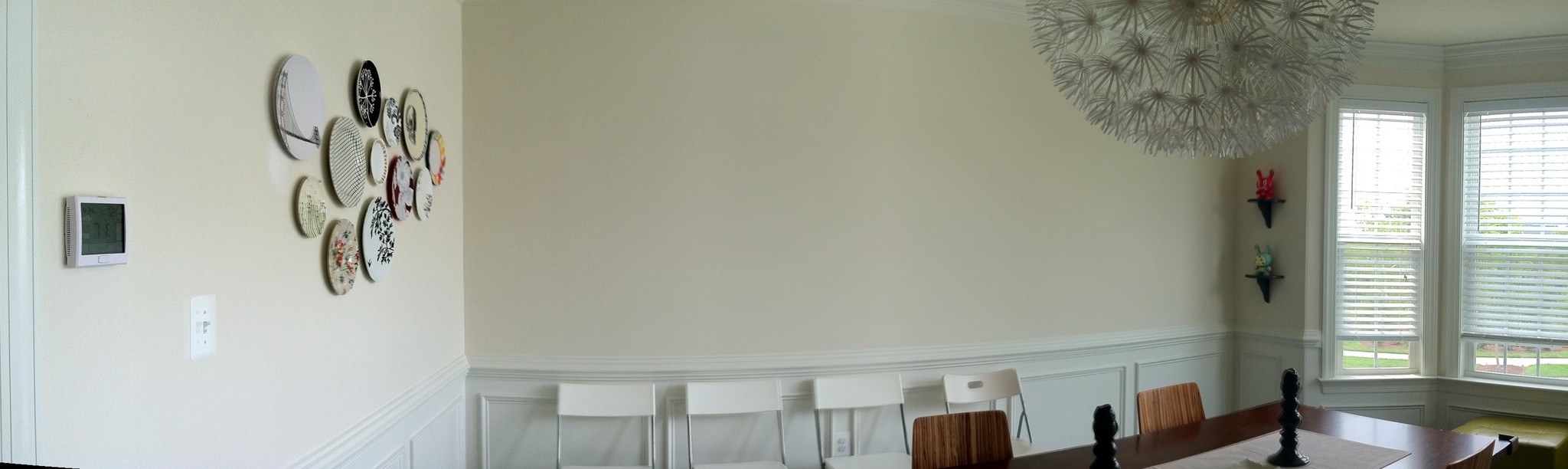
[525,365,1219,469]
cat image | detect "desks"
[957,394,1518,469]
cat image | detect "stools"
[1448,415,1567,469]
